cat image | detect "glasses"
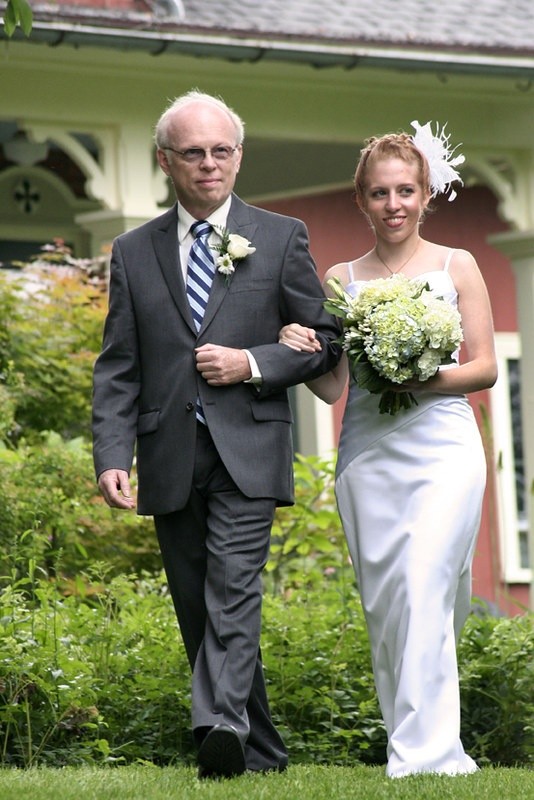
[161,143,237,163]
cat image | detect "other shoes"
[199,723,244,780]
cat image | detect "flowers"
[206,222,255,287]
[411,120,466,201]
[323,275,465,417]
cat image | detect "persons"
[92,92,343,780]
[278,133,499,779]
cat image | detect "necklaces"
[376,237,421,280]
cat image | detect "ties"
[185,221,215,425]
